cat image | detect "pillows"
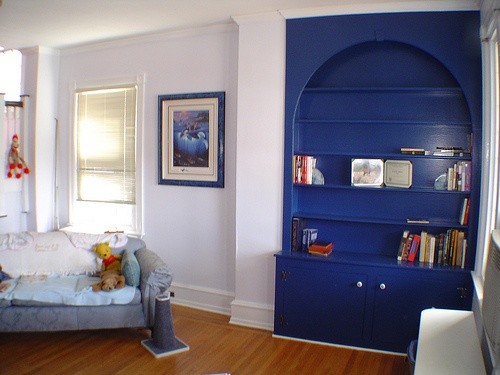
[120,249,140,287]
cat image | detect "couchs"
[0,231,189,358]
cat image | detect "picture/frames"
[384,160,412,188]
[158,91,225,189]
[350,158,384,187]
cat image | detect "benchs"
[413,309,486,375]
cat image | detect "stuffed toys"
[94,242,122,273]
[93,270,125,291]
[7,135,30,178]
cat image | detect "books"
[396,228,467,269]
[431,146,467,157]
[401,147,425,155]
[459,198,470,225]
[292,217,334,257]
[447,160,471,192]
[466,133,473,157]
[407,218,429,223]
[292,155,317,185]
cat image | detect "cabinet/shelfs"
[273,10,483,357]
